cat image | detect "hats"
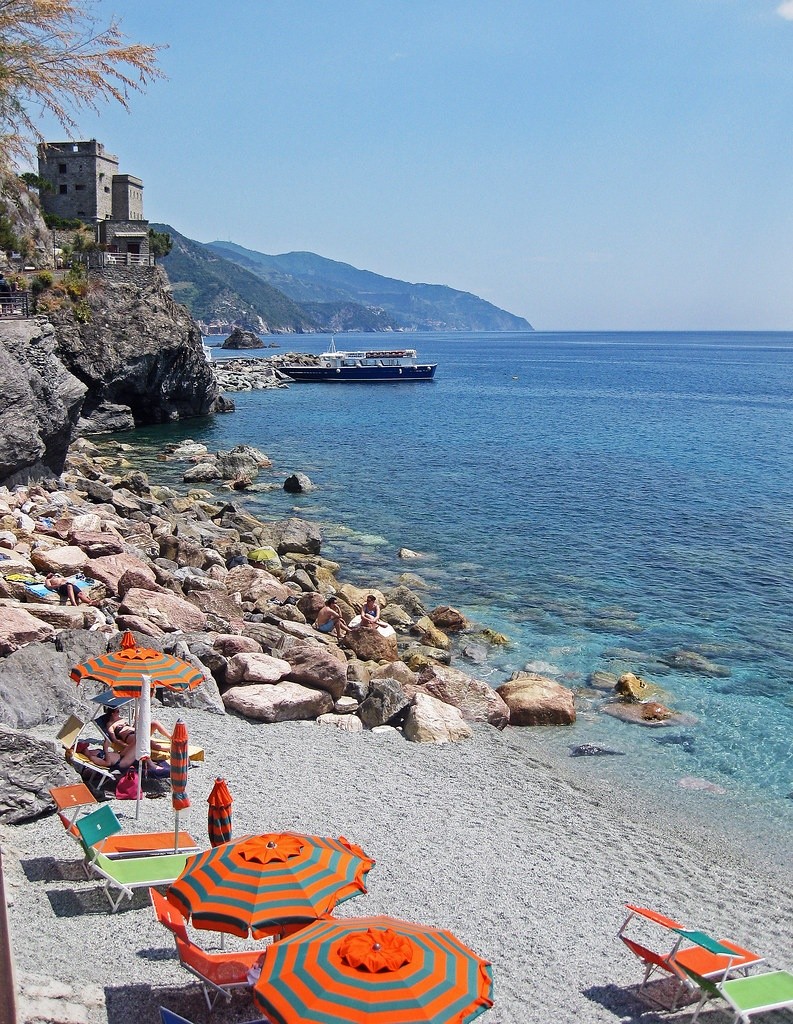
[76,741,89,752]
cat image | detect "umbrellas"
[251,916,494,1024]
[70,646,205,726]
[136,674,151,821]
[171,718,188,854]
[121,628,134,726]
[207,776,233,950]
[166,831,375,941]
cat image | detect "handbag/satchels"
[116,766,143,800]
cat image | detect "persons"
[45,572,98,607]
[13,276,22,292]
[107,254,111,260]
[67,260,72,268]
[318,597,352,638]
[74,709,171,770]
[0,274,13,312]
[58,256,63,269]
[361,595,388,628]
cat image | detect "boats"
[272,333,439,384]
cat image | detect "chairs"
[55,712,171,791]
[49,782,202,882]
[157,1005,270,1024]
[615,903,768,1013]
[148,885,283,1014]
[666,926,793,1024]
[89,690,206,771]
[75,803,199,914]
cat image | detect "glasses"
[334,602,337,604]
[113,709,119,713]
[367,599,372,602]
